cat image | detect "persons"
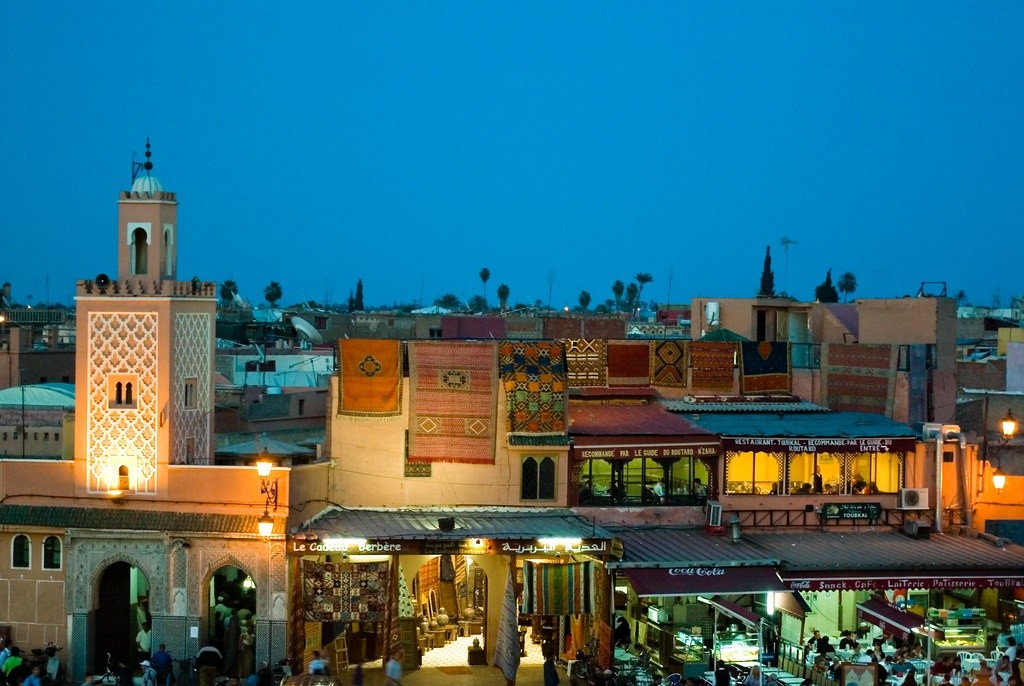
[649,675,707,686]
[194,595,329,686]
[715,659,778,686]
[578,475,626,503]
[353,651,402,686]
[695,478,706,497]
[852,476,877,494]
[543,652,560,686]
[768,482,778,495]
[654,475,666,502]
[800,625,1024,686]
[0,639,65,686]
[615,610,624,629]
[81,660,135,686]
[136,598,172,686]
[795,465,839,495]
[587,665,637,686]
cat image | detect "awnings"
[574,434,720,461]
[621,567,1024,601]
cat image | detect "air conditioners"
[897,488,930,510]
[707,500,722,527]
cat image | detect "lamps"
[989,408,1016,495]
[255,461,278,536]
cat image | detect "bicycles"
[99,652,122,686]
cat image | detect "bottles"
[416,607,449,636]
[473,639,479,648]
[464,604,475,621]
[814,640,817,650]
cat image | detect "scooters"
[574,642,617,686]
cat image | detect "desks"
[961,659,996,680]
[886,673,926,686]
[417,621,471,656]
[781,636,935,686]
[930,675,964,686]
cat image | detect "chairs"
[911,662,926,674]
[956,651,1005,683]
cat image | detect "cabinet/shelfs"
[712,630,760,662]
[669,632,709,678]
[929,620,988,649]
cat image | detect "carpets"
[300,337,917,620]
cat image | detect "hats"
[139,660,150,666]
[218,596,224,601]
[953,657,961,662]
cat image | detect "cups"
[846,644,849,650]
